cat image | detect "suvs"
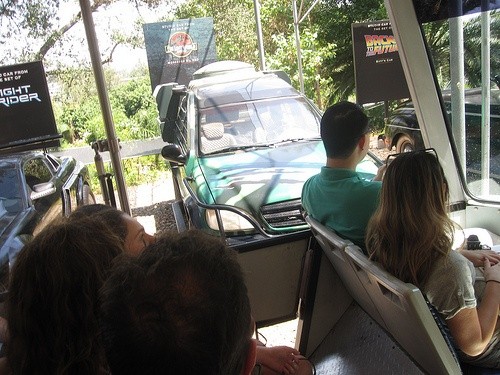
[151,59,384,246]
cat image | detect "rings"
[292,359,294,363]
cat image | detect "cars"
[0,149,98,286]
[382,86,500,190]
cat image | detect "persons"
[0,204,154,375]
[97,228,257,375]
[366,148,500,375]
[302,102,390,255]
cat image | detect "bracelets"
[486,279,500,284]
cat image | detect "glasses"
[385,148,439,168]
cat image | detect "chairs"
[200,122,238,155]
[301,210,464,375]
